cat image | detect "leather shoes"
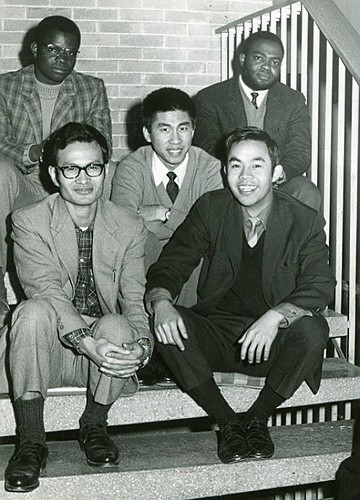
[77,425,121,468]
[3,440,48,493]
[215,417,274,463]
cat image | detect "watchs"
[162,208,171,225]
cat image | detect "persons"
[144,125,338,467]
[189,32,325,216]
[109,86,227,389]
[0,16,113,339]
[4,122,156,493]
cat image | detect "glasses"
[36,42,80,58]
[54,164,105,179]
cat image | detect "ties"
[247,216,261,247]
[250,92,259,110]
[165,171,179,203]
